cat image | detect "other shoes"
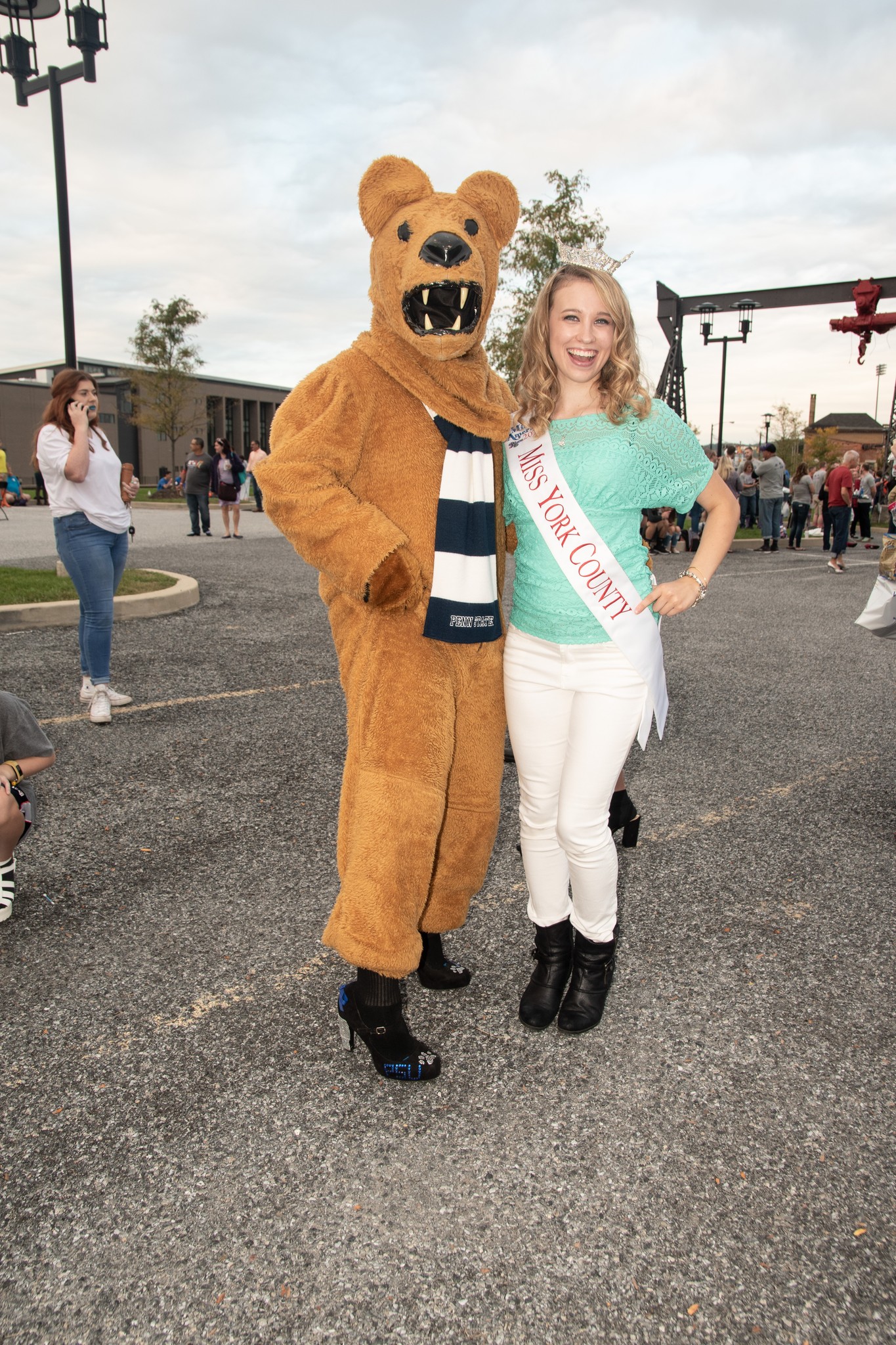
[754,527,880,554]
[186,531,242,539]
[252,508,265,512]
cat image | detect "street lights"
[2,0,109,373]
[761,413,775,443]
[875,362,887,422]
[689,296,765,457]
[709,420,735,450]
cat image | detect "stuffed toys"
[252,154,654,1080]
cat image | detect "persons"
[0,689,56,924]
[0,439,30,508]
[502,261,896,1036]
[157,470,184,494]
[30,369,142,724]
[243,440,269,513]
[186,436,245,539]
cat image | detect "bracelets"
[686,567,707,590]
[2,760,24,786]
[679,566,708,609]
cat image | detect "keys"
[128,526,135,543]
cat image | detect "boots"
[518,914,574,1030]
[558,923,621,1033]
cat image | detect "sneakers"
[89,684,112,722]
[665,548,671,554]
[670,548,680,553]
[653,546,669,555]
[80,686,131,706]
[649,549,659,556]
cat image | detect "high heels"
[0,858,16,922]
[836,561,846,570]
[827,562,844,574]
[337,981,441,1081]
[416,930,470,989]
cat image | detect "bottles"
[120,463,133,501]
[224,460,228,471]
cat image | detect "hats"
[760,443,776,453]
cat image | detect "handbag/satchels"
[218,480,238,501]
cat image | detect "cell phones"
[65,398,88,415]
